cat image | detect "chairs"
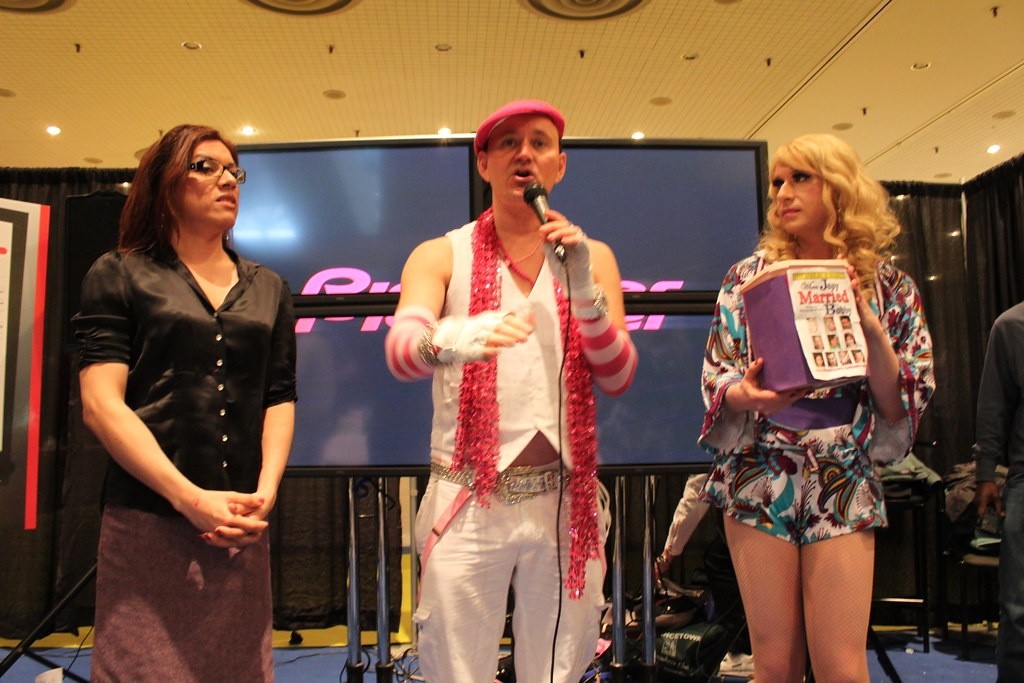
[955,509,999,650]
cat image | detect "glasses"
[186,159,246,184]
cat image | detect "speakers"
[63,190,129,351]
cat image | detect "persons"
[807,316,865,366]
[385,99,639,683]
[72,124,297,683]
[697,134,937,683]
[652,472,735,624]
[974,299,1024,683]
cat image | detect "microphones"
[523,181,567,263]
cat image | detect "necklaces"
[499,237,542,284]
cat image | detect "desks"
[869,497,932,655]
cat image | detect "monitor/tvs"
[217,139,775,476]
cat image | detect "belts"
[431,462,566,505]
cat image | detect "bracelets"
[656,556,669,567]
[417,326,439,368]
[572,290,608,319]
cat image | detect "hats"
[473,99,565,156]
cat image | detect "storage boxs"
[738,260,870,390]
